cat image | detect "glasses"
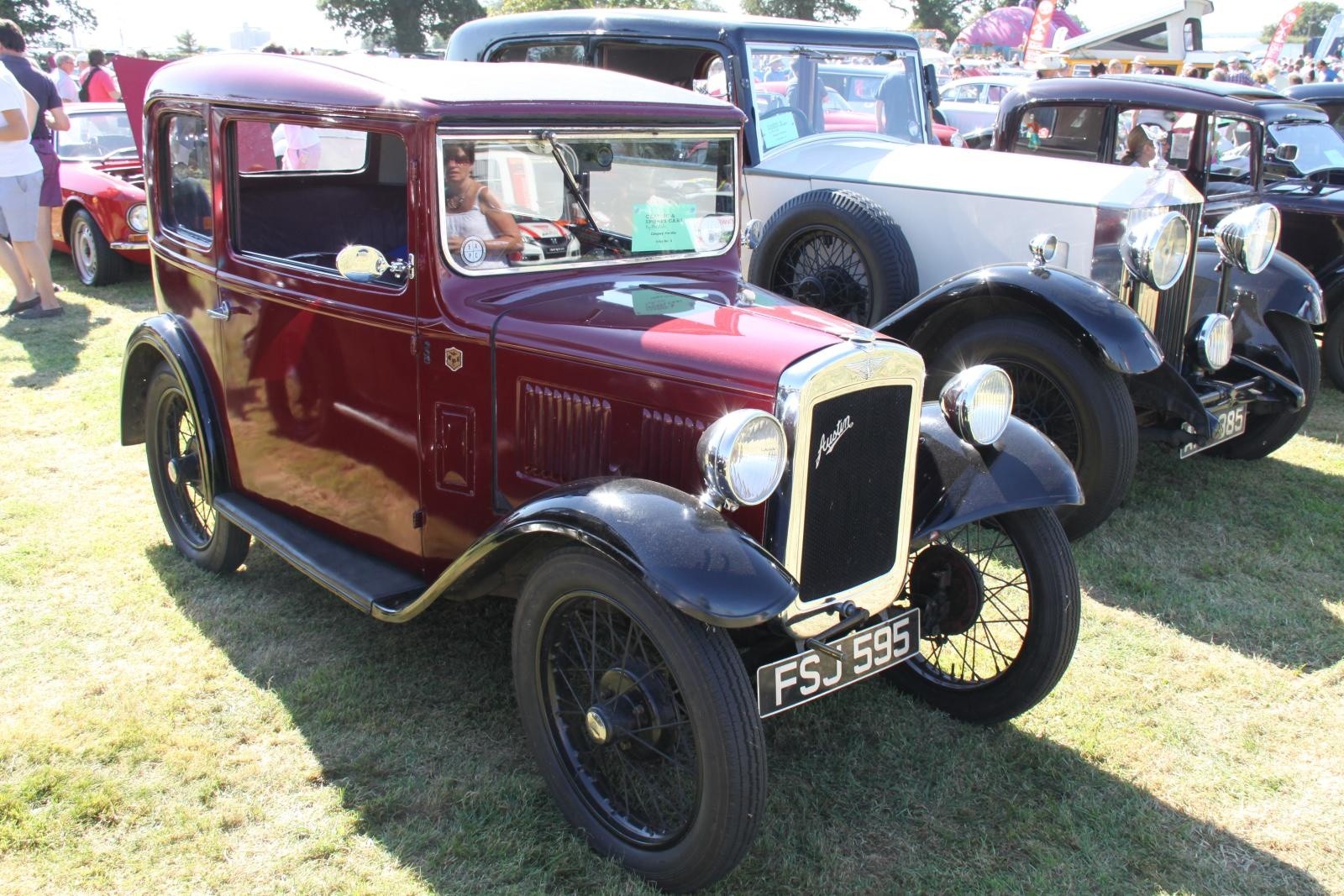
[442,156,472,166]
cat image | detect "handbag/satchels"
[77,68,101,101]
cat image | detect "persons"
[0,18,70,292]
[1180,63,1199,78]
[764,55,790,82]
[974,51,1020,66]
[291,47,317,55]
[443,143,525,270]
[785,54,828,133]
[876,60,932,140]
[1120,123,1171,167]
[953,57,965,79]
[1205,44,1344,93]
[1027,121,1041,150]
[0,61,65,321]
[284,124,321,169]
[1089,56,1175,152]
[389,46,399,57]
[46,49,149,144]
[852,57,871,65]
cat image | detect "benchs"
[240,184,406,267]
[60,142,92,158]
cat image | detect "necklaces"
[445,189,465,209]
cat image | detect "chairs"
[93,132,135,155]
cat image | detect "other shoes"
[0,296,41,315]
[12,304,65,317]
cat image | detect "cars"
[684,0,1344,396]
[443,9,1329,543]
[116,52,1089,896]
[43,55,212,287]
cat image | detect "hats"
[767,54,782,64]
[1130,56,1149,64]
[1034,54,1065,70]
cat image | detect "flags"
[1024,0,1057,63]
